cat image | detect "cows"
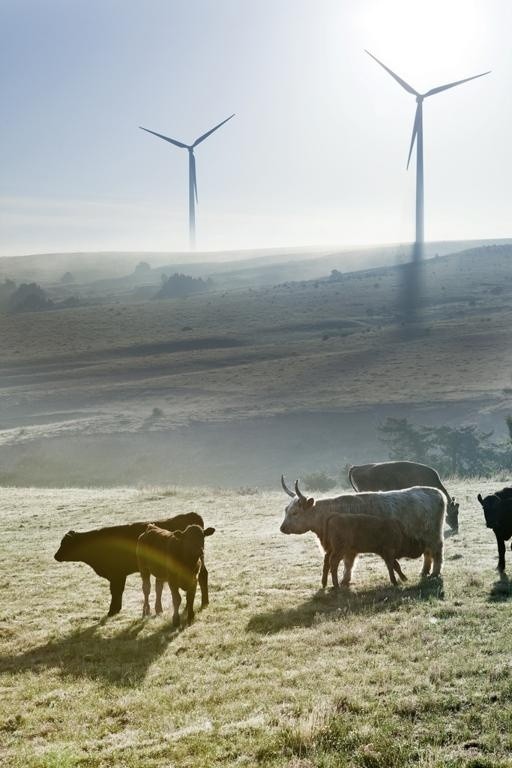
[322,512,427,590]
[54,512,209,617]
[349,461,459,532]
[136,524,215,628]
[280,475,448,589]
[477,487,512,571]
